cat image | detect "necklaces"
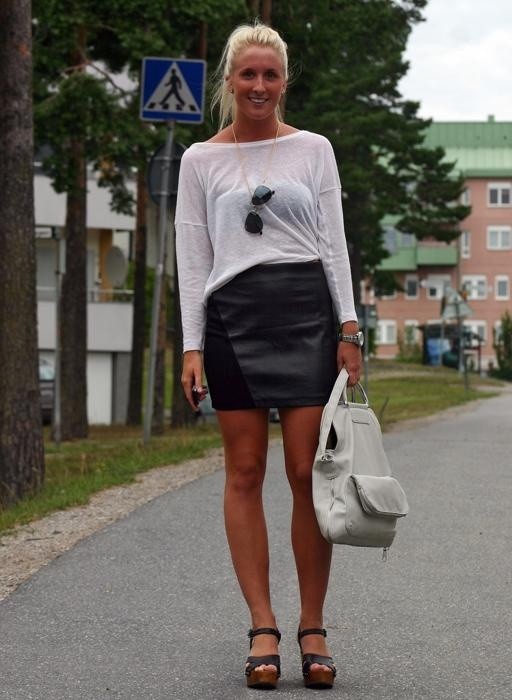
[229,120,280,200]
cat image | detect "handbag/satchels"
[312,357,409,556]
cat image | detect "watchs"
[336,331,365,347]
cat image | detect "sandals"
[298,623,336,688]
[244,627,281,690]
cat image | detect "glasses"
[244,183,275,235]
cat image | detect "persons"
[173,21,367,690]
